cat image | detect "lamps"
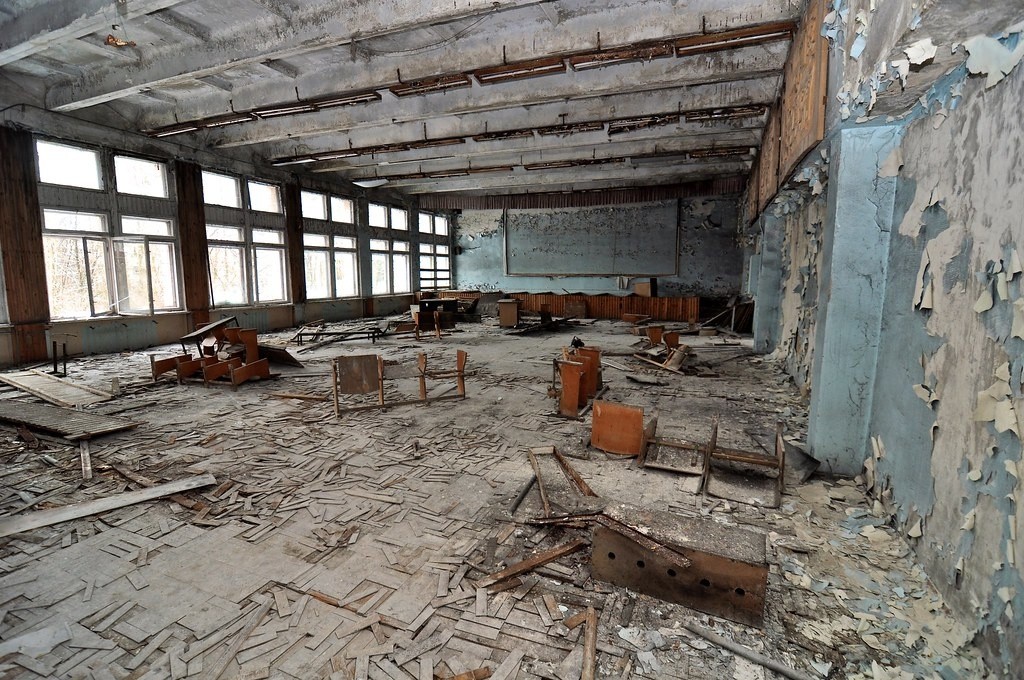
[155,30,793,139]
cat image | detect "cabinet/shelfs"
[498,299,521,328]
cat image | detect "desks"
[419,298,459,332]
[180,316,239,358]
